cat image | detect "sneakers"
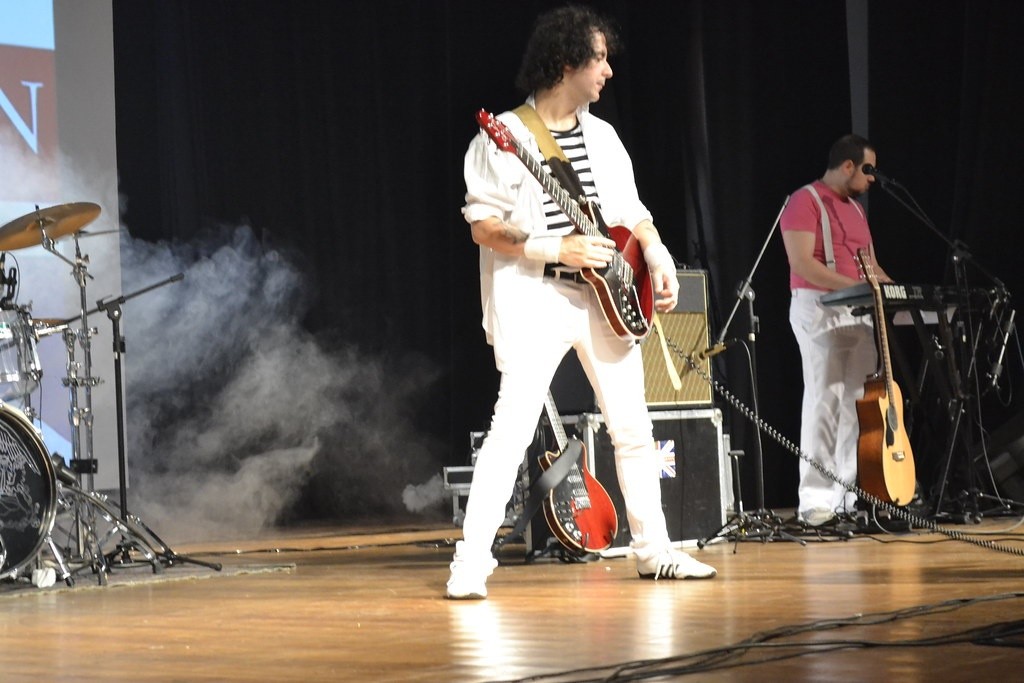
[447,540,498,599]
[636,548,716,581]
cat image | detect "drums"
[0,310,44,402]
[0,400,58,580]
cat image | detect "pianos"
[820,280,1013,532]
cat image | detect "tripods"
[695,196,854,550]
[39,222,221,586]
[880,182,1023,524]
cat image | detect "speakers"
[641,269,714,410]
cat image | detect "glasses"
[860,164,879,176]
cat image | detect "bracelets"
[523,231,563,265]
[644,242,676,275]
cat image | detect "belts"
[543,268,588,283]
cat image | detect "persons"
[446,7,720,599]
[778,133,897,533]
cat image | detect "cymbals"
[0,201,102,251]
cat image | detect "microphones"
[862,163,902,188]
[691,338,738,366]
[0,253,5,297]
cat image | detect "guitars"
[851,246,916,506]
[538,388,619,556]
[475,107,656,343]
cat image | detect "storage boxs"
[444,409,727,557]
